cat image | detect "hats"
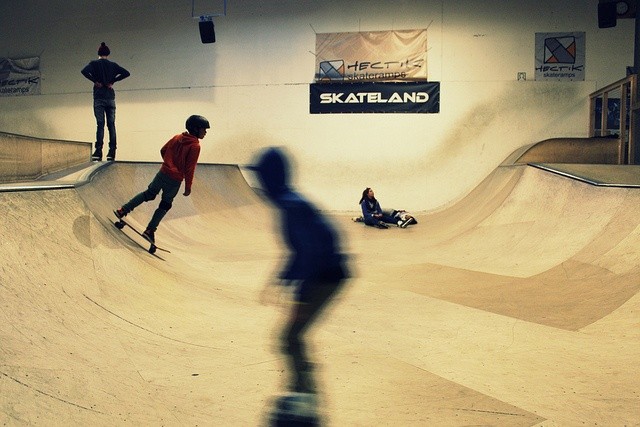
[97,42,110,56]
[242,148,288,175]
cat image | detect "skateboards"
[267,408,323,427]
[115,218,171,254]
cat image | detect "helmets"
[186,115,210,129]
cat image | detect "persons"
[81,41,130,161]
[351,208,417,228]
[243,147,354,426]
[112,115,210,243]
[359,188,389,229]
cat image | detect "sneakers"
[92,149,102,159]
[106,149,115,158]
[142,229,155,245]
[400,218,413,228]
[116,208,125,218]
[391,210,401,219]
